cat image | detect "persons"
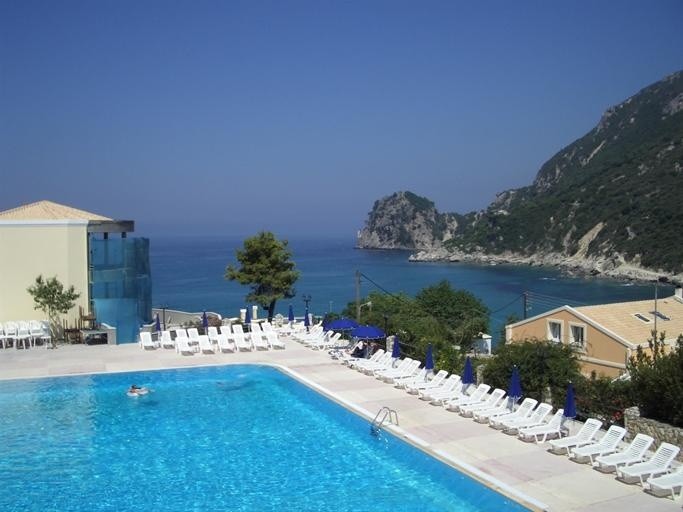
[128,385,137,393]
[202,312,208,335]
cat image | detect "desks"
[79,329,108,345]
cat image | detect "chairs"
[488,397,539,430]
[569,425,626,465]
[617,441,680,487]
[548,418,603,455]
[282,318,506,417]
[472,395,522,424]
[518,408,570,444]
[593,433,654,473]
[502,402,553,435]
[139,320,285,353]
[646,461,681,502]
[0,306,97,351]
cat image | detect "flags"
[245,308,250,333]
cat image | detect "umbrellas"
[304,308,309,332]
[323,318,361,349]
[155,313,161,341]
[288,304,294,329]
[425,343,434,382]
[350,325,384,359]
[462,356,474,393]
[507,365,523,413]
[391,333,401,367]
[562,381,576,436]
